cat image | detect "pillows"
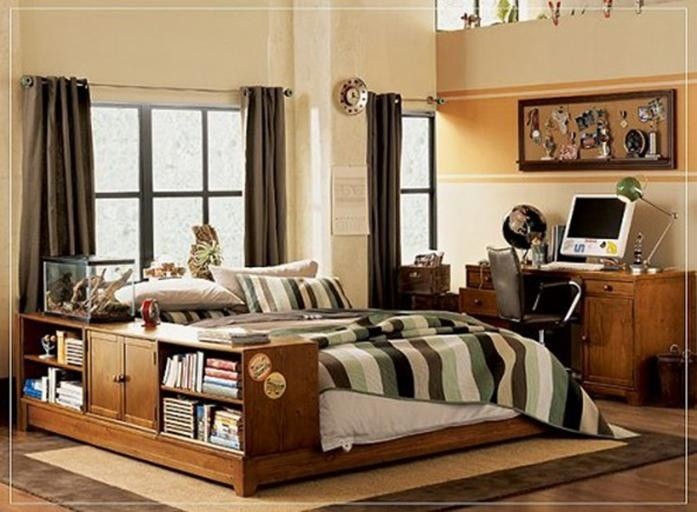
[113,258,355,313]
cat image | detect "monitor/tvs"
[559,194,637,263]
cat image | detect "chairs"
[485,244,587,390]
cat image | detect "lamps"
[617,175,679,273]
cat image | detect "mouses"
[600,266,619,272]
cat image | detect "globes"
[502,204,547,265]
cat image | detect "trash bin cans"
[656,344,697,410]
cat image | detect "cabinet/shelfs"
[569,277,697,407]
[459,269,510,331]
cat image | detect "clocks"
[338,78,369,115]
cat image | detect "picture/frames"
[518,89,676,171]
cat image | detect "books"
[550,224,588,264]
[195,325,271,346]
[20,330,84,410]
[159,349,245,452]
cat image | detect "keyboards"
[540,261,604,272]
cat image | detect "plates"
[623,129,646,156]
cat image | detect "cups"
[531,245,548,266]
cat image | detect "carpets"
[0,413,696,512]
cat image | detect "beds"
[105,308,589,498]
